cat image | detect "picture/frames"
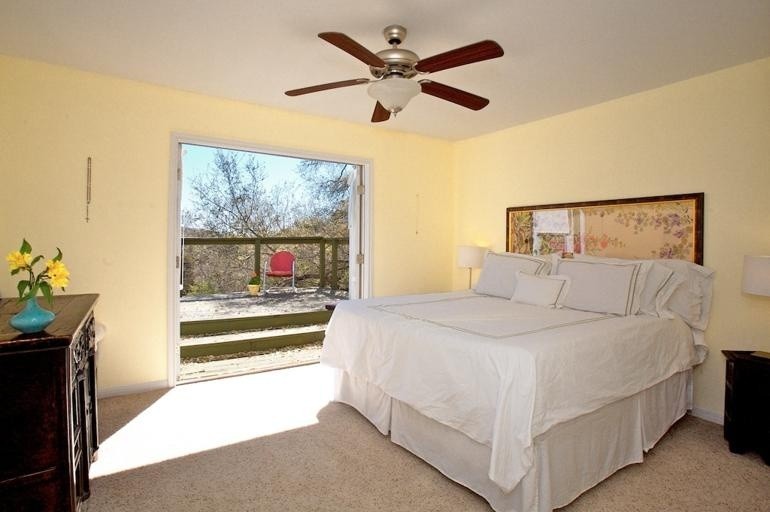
[504,189,704,267]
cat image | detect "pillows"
[469,246,718,336]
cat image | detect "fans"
[284,23,507,124]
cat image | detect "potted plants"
[246,276,261,296]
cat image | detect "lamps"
[455,246,488,291]
[362,70,424,119]
[738,253,770,300]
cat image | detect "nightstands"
[718,347,770,468]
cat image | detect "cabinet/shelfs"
[0,293,105,512]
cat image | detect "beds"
[318,290,709,511]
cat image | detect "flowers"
[5,234,69,305]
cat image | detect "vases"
[8,292,55,334]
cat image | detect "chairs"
[261,251,297,294]
